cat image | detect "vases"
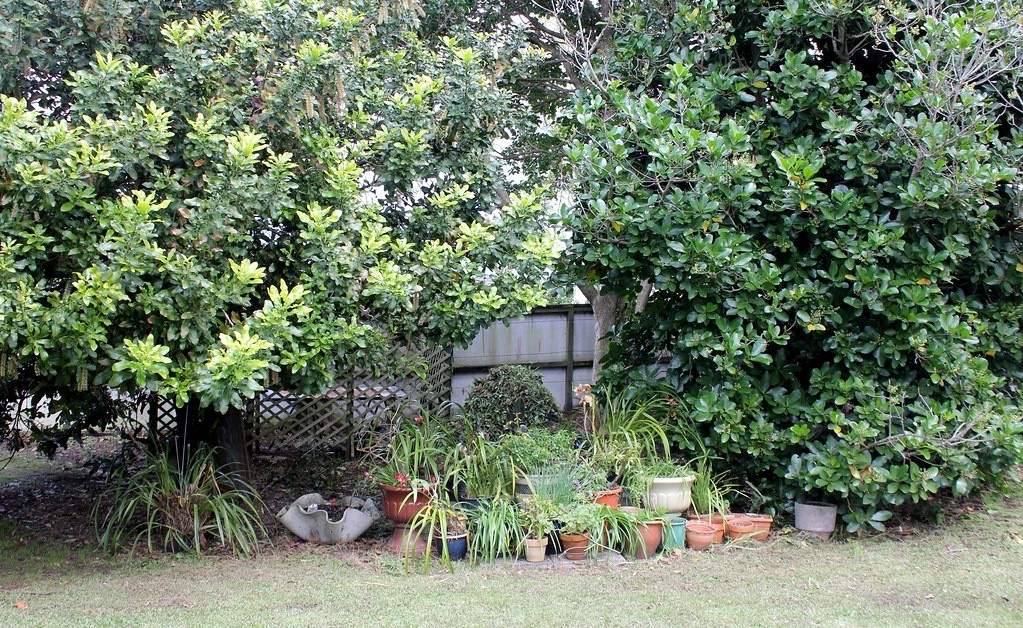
[378,483,431,554]
[795,501,838,541]
[275,493,382,543]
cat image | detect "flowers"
[361,470,436,496]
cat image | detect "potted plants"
[357,362,774,563]
